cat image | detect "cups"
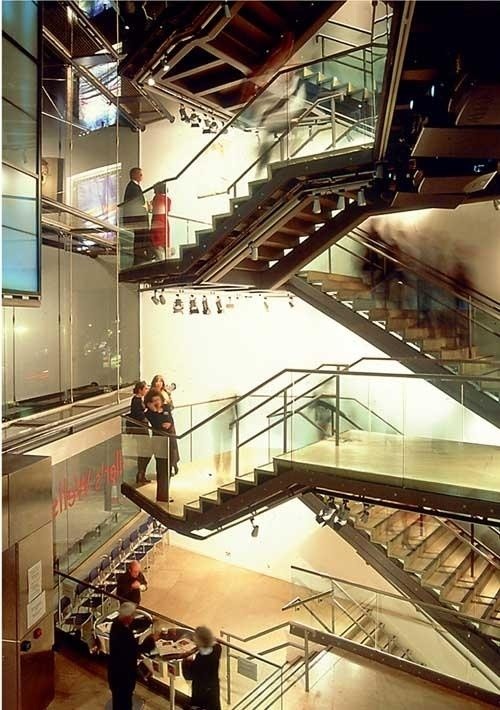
[162,628,168,635]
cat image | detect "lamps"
[309,492,373,535]
[306,183,371,215]
[247,514,265,540]
[144,290,300,319]
[177,101,254,140]
[145,49,171,88]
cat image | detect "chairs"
[54,510,174,640]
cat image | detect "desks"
[138,627,205,709]
[87,602,158,658]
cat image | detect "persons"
[361,220,473,351]
[128,374,179,501]
[117,560,147,609]
[123,167,151,262]
[182,628,222,710]
[146,182,170,261]
[108,603,155,710]
